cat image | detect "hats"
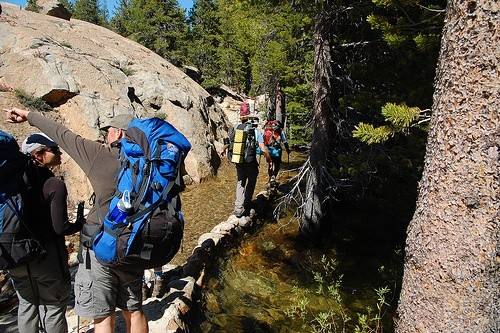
[21,133,58,155]
[245,112,259,124]
[98,113,135,130]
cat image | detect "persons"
[256,123,290,187]
[4,133,86,333]
[4,107,150,333]
[230,117,272,217]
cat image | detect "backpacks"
[264,121,284,157]
[91,116,191,272]
[227,123,256,163]
[0,130,56,267]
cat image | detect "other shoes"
[142,286,148,300]
[152,279,164,295]
